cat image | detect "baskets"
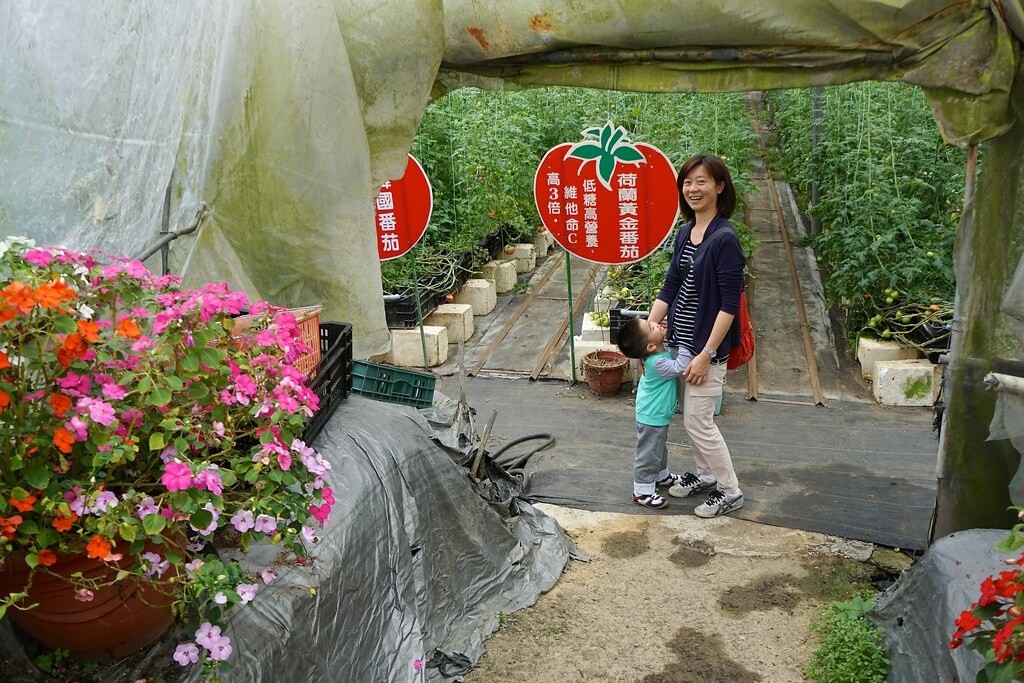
[235,320,354,455]
[350,359,436,408]
[215,304,321,390]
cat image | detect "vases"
[582,349,630,397]
[2,513,187,657]
[384,225,508,330]
[916,314,953,363]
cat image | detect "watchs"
[703,347,718,358]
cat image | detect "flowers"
[0,233,334,675]
[950,506,1024,683]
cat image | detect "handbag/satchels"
[727,287,755,369]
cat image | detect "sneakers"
[657,473,685,488]
[694,490,744,518]
[633,491,669,509]
[669,472,717,498]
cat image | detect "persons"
[617,317,695,509]
[637,154,747,518]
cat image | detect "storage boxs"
[351,361,437,408]
[230,309,353,454]
[225,304,320,382]
